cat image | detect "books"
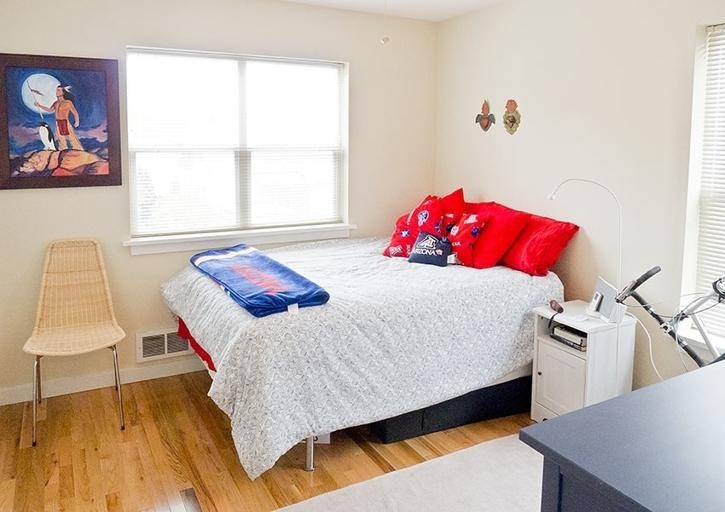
[553,324,587,347]
[551,333,587,352]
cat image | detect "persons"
[35,84,84,150]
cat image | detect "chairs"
[22,237,127,447]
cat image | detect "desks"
[517,358,725,512]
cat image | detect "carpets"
[269,433,544,512]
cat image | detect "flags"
[28,81,44,96]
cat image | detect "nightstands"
[530,298,639,426]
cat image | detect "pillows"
[381,187,579,279]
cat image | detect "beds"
[162,236,564,482]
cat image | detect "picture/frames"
[0,52,122,190]
